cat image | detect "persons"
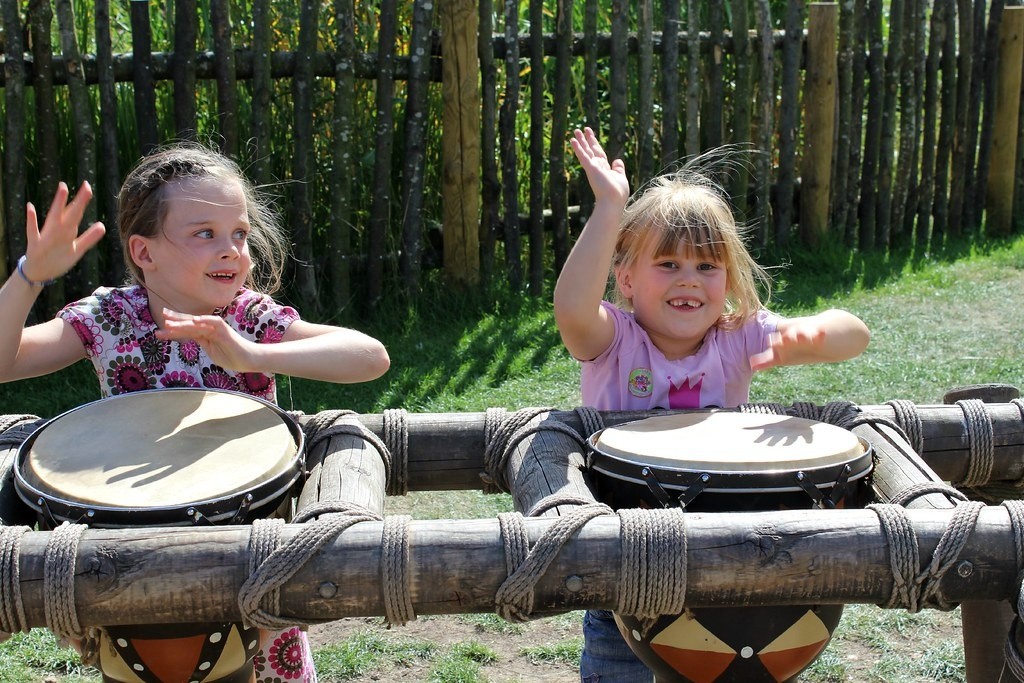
[0,149,391,683]
[554,125,870,683]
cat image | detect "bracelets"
[18,255,54,287]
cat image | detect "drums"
[13,385,313,683]
[584,409,874,683]
